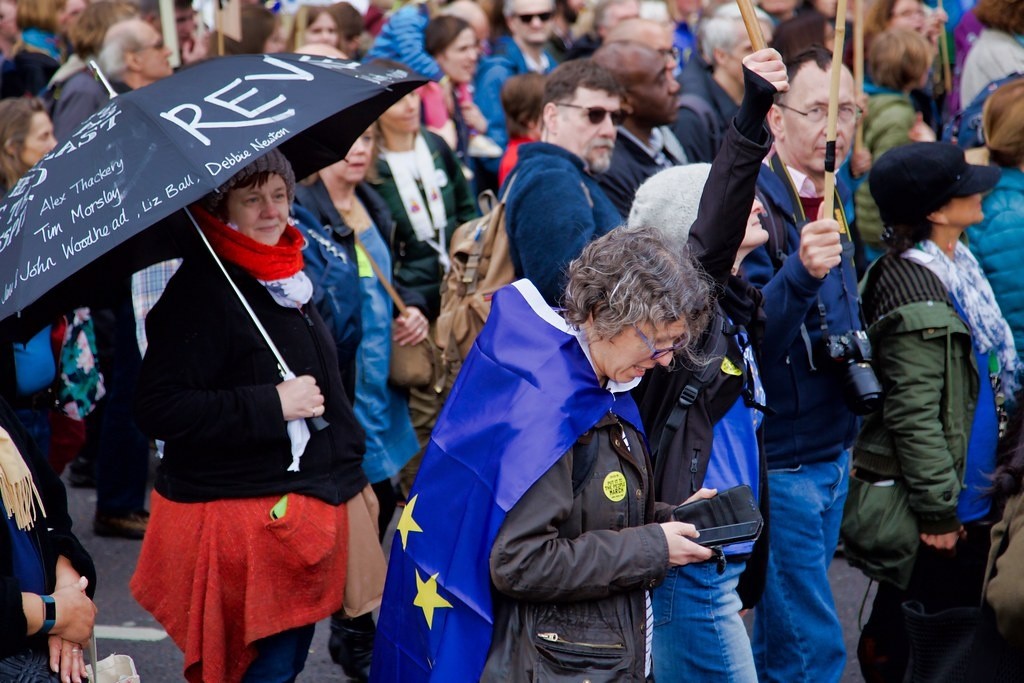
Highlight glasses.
[627,316,688,360]
[513,10,558,24]
[658,45,684,66]
[554,101,628,128]
[775,99,864,131]
[126,38,168,55]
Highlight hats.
[205,147,298,215]
[868,142,1002,216]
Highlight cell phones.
[684,520,763,546]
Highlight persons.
[0,0,1024,683]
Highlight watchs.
[40,596,56,633]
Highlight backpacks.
[434,165,594,393]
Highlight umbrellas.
[0,52,435,432]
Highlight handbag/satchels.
[904,597,1013,683]
[531,592,636,683]
[79,627,141,683]
[386,317,433,387]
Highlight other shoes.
[93,503,151,537]
[329,608,378,683]
[68,461,91,487]
[467,133,507,157]
[427,122,459,153]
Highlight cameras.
[817,329,886,416]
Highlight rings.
[71,650,79,652]
[417,328,423,334]
[313,408,315,417]
